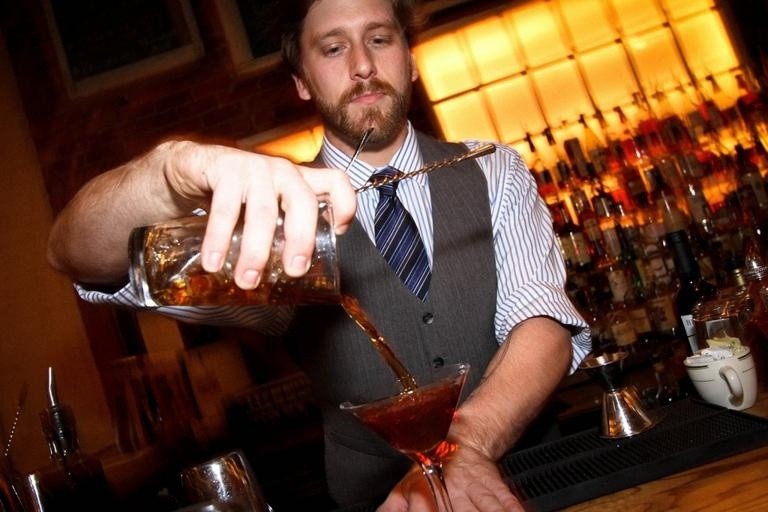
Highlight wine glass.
[339,362,471,512]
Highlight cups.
[167,448,270,511]
[683,347,758,411]
[127,193,341,309]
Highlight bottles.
[39,404,116,510]
[524,71,765,368]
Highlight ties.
[367,165,432,306]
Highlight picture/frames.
[209,0,296,80]
[40,1,207,102]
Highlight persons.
[49,0,592,512]
